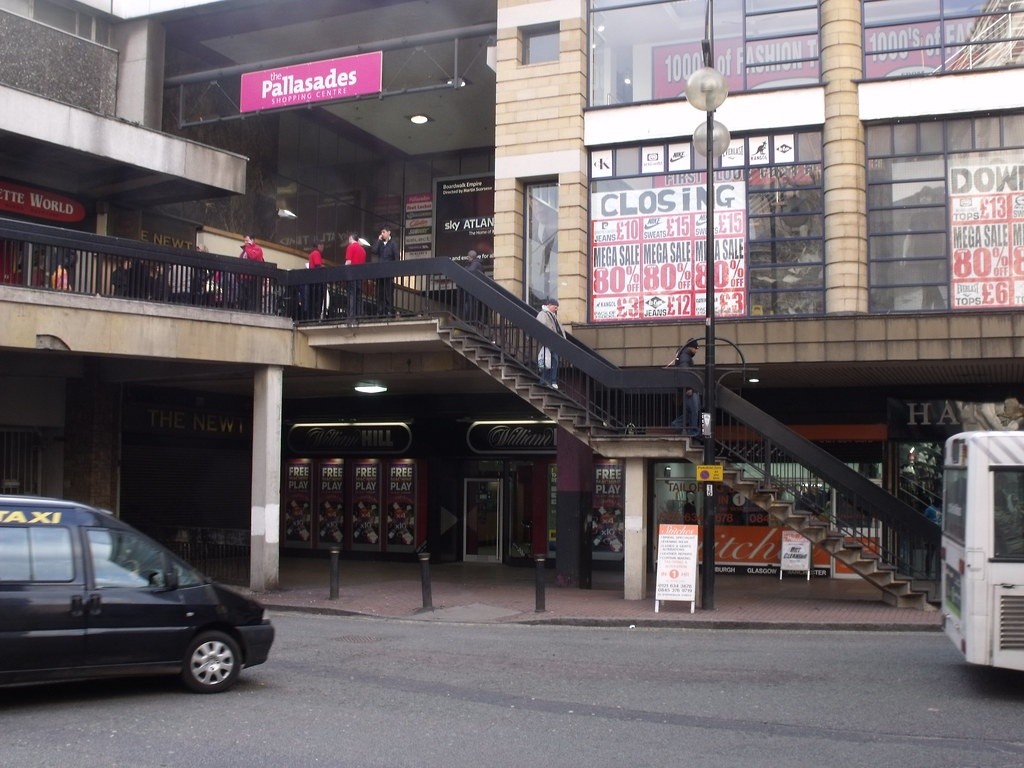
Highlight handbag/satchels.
[538,346,551,372]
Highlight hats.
[686,338,700,349]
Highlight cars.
[0,493,276,695]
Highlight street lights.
[684,38,731,613]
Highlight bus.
[938,428,1024,677]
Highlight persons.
[923,494,941,577]
[52,263,71,291]
[535,299,566,389]
[113,258,195,304]
[220,273,239,309]
[240,233,264,312]
[308,242,324,318]
[672,338,700,438]
[371,227,399,319]
[261,277,273,313]
[345,233,366,316]
[466,249,485,274]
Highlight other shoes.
[689,431,702,440]
[377,311,386,319]
[671,422,683,434]
[552,383,558,389]
[388,310,397,318]
